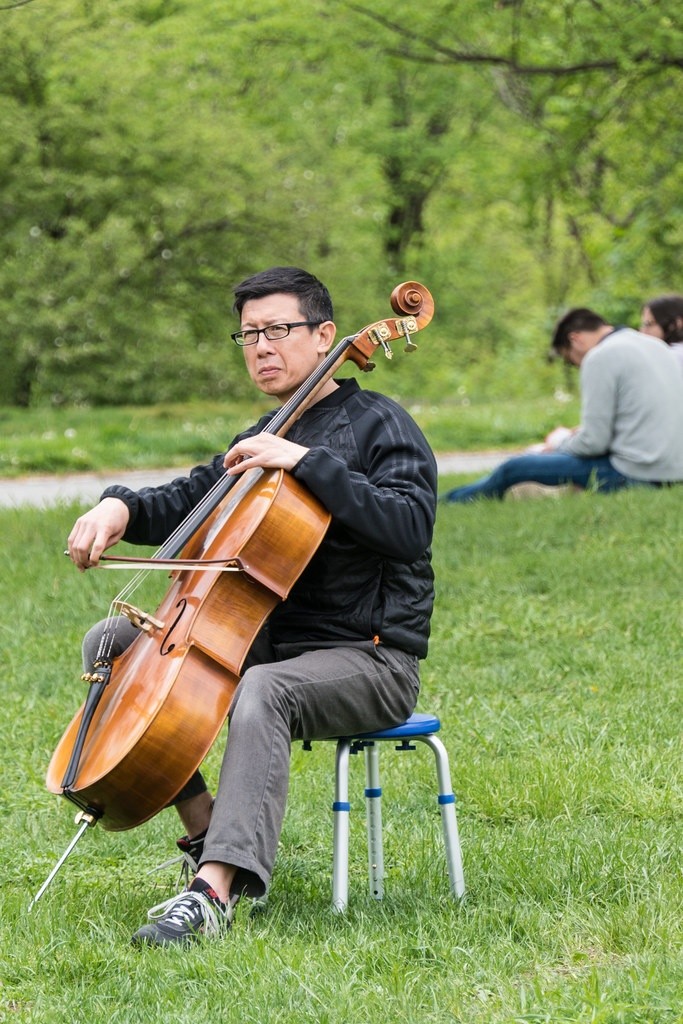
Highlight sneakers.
[130,877,239,950]
[147,797,240,909]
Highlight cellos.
[21,281,436,918]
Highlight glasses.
[564,344,574,369]
[639,322,658,329]
[230,321,321,346]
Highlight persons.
[440,296,683,504]
[68,267,438,951]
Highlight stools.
[332,712,467,914]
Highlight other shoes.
[504,482,584,503]
